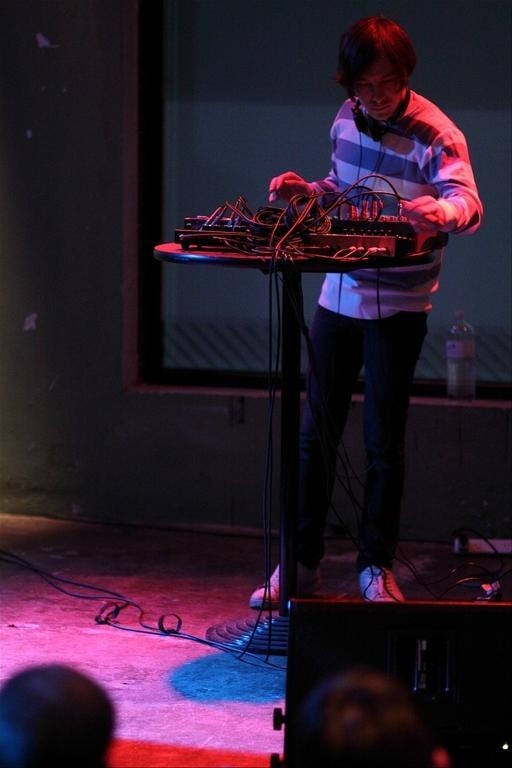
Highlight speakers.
[273,594,512,767]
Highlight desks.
[150,237,436,658]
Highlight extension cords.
[454,538,512,556]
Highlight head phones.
[352,85,411,142]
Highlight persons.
[247,17,489,612]
[302,667,431,767]
[0,664,117,768]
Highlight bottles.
[444,309,477,402]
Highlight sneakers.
[249,559,322,608]
[360,566,404,602]
